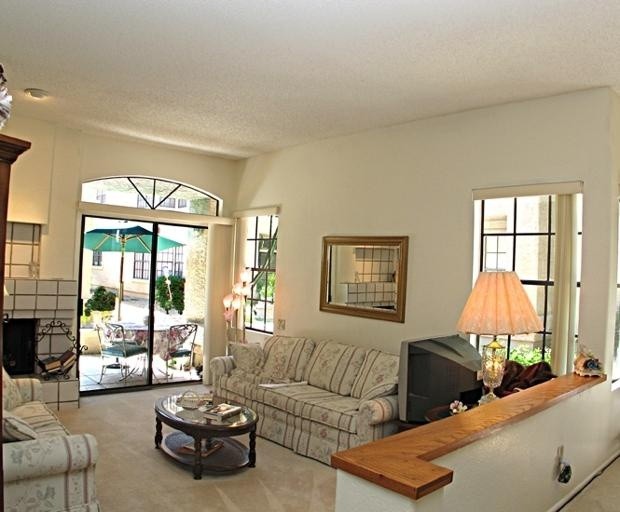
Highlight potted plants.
[88,282,116,320]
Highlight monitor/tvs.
[397,335,483,423]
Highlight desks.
[423,402,479,423]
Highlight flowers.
[450,400,467,416]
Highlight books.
[39,349,76,375]
[179,436,223,457]
[205,402,242,421]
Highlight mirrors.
[319,235,409,325]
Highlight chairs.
[95,318,197,386]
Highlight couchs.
[2,367,100,511]
[209,333,400,467]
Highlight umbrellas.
[83,225,186,320]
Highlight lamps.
[456,267,545,406]
[221,268,256,334]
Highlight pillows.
[224,337,265,373]
[301,340,361,396]
[354,374,398,411]
[347,347,402,397]
[260,332,316,383]
